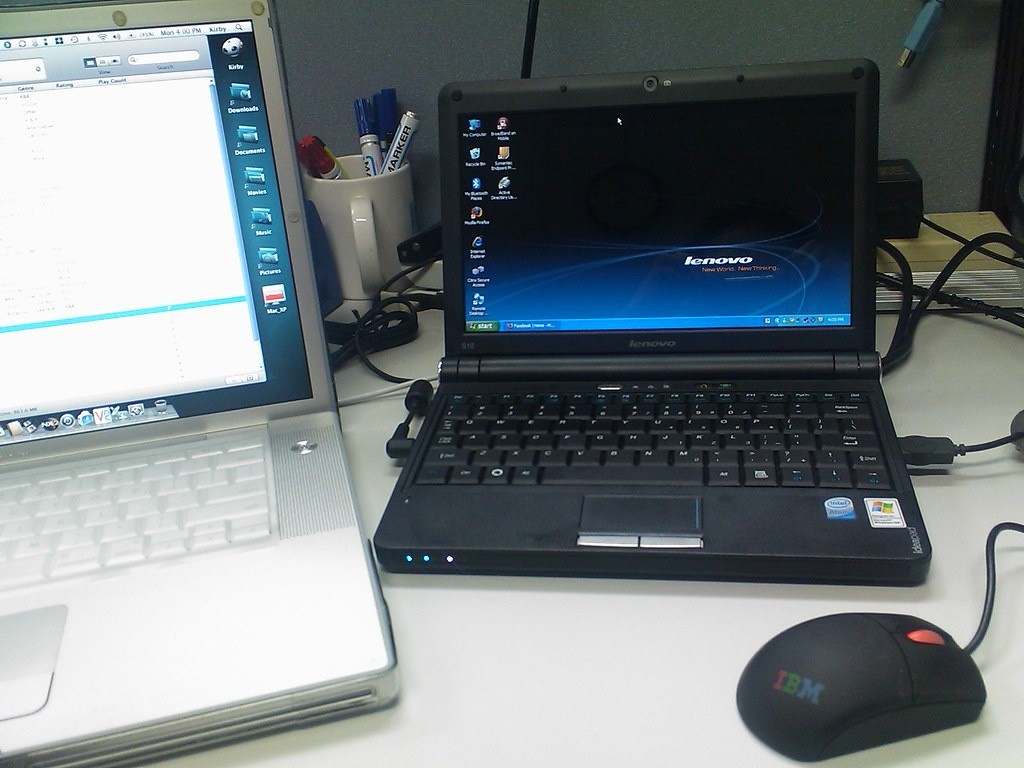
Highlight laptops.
[0,0,399,768]
[374,57,931,587]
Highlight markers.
[299,86,420,181]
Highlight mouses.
[736,612,987,763]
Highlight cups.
[302,154,417,300]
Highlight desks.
[131,260,1024,768]
